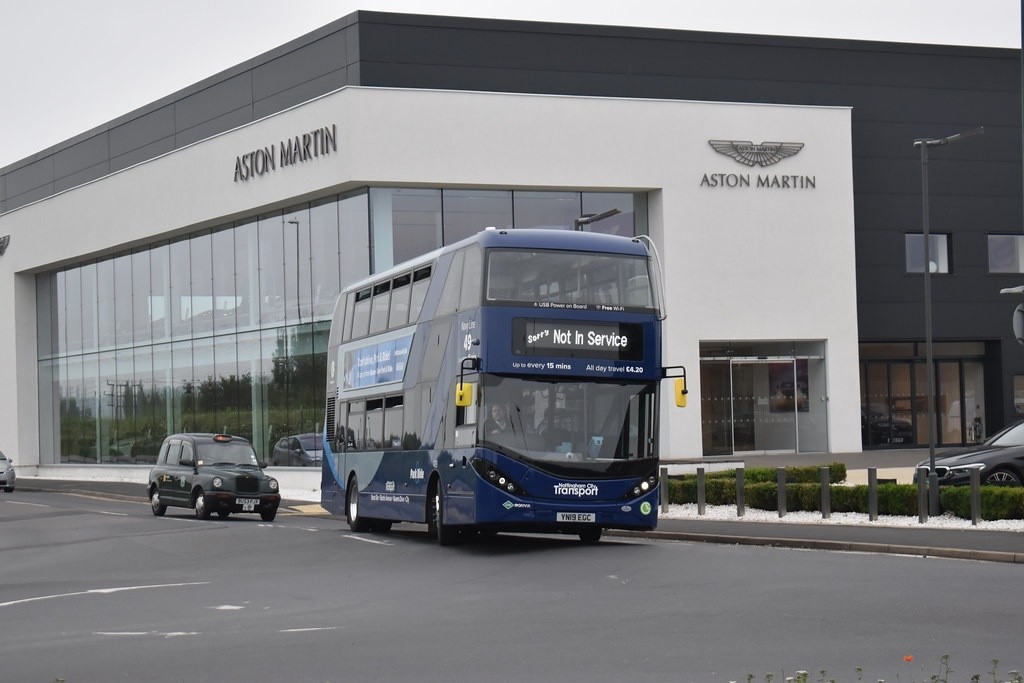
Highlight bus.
[320,229,690,548]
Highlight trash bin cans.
[877,479,896,484]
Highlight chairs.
[484,407,585,455]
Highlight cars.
[915,417,1024,487]
[0,451,17,493]
[272,433,324,467]
[147,433,281,522]
[861,405,916,447]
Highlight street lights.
[913,128,986,518]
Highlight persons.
[181,423,192,433]
[484,405,513,433]
[241,424,248,433]
[269,422,293,433]
